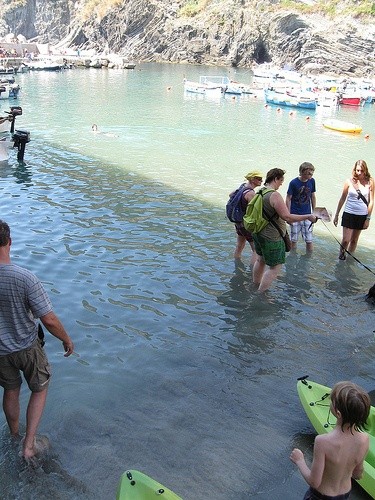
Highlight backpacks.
[243,187,276,235]
[226,183,255,223]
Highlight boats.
[0,48,135,100]
[116,469,184,500]
[323,118,363,135]
[297,374,374,500]
[177,55,375,110]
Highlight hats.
[245,171,265,178]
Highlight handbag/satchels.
[283,230,292,252]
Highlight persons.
[250,168,318,292]
[289,380,370,500]
[333,159,374,260]
[285,162,316,254]
[90,124,99,134]
[0,220,75,463]
[231,172,264,264]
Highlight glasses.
[249,176,262,182]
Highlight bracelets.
[366,216,371,220]
